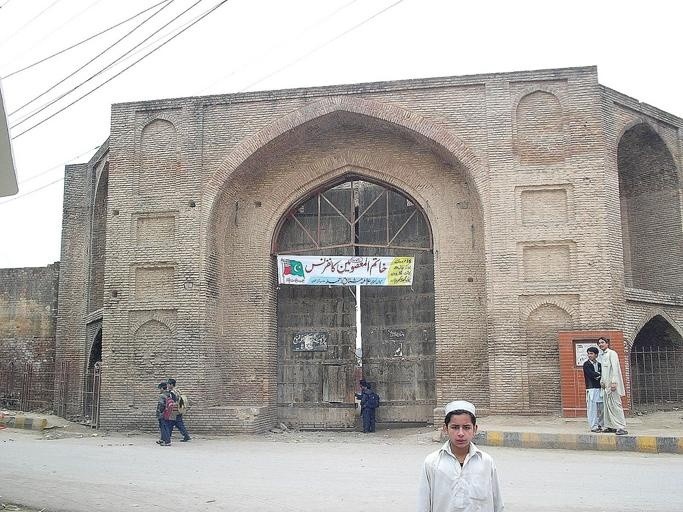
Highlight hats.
[445,400,476,416]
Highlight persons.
[168,378,192,442]
[415,400,506,512]
[355,380,376,432]
[583,347,604,432]
[155,383,177,446]
[597,337,629,434]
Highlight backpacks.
[176,391,188,414]
[366,393,379,408]
[160,395,178,421]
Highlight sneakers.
[156,439,170,446]
[180,437,190,442]
[592,425,627,434]
